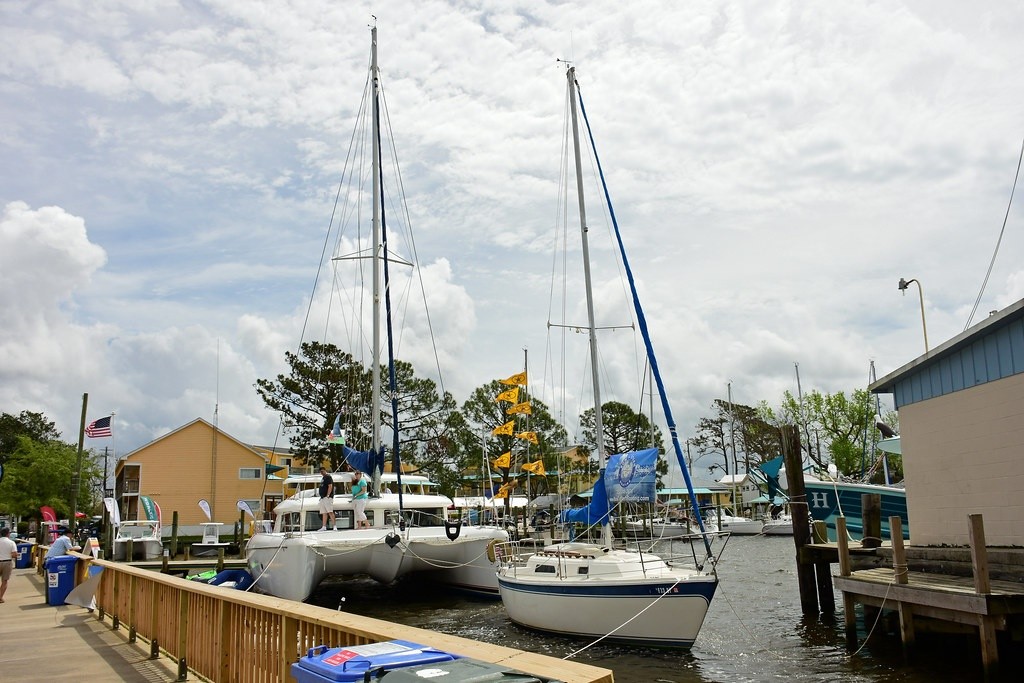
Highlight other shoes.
[0,599,4,603]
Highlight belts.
[0,560,12,562]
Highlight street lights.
[897,277,929,353]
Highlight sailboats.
[488,348,571,544]
[494,50,722,651]
[617,350,704,539]
[706,382,763,534]
[241,15,508,610]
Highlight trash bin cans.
[290,640,455,683]
[357,658,544,683]
[44,555,78,606]
[15,543,34,568]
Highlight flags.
[604,447,657,505]
[140,496,158,529]
[103,498,120,527]
[85,416,113,439]
[485,483,499,500]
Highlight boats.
[763,518,815,537]
[798,475,909,543]
[113,518,163,562]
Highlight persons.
[349,470,371,530]
[317,467,338,531]
[43,530,82,561]
[381,483,392,494]
[0,526,18,604]
